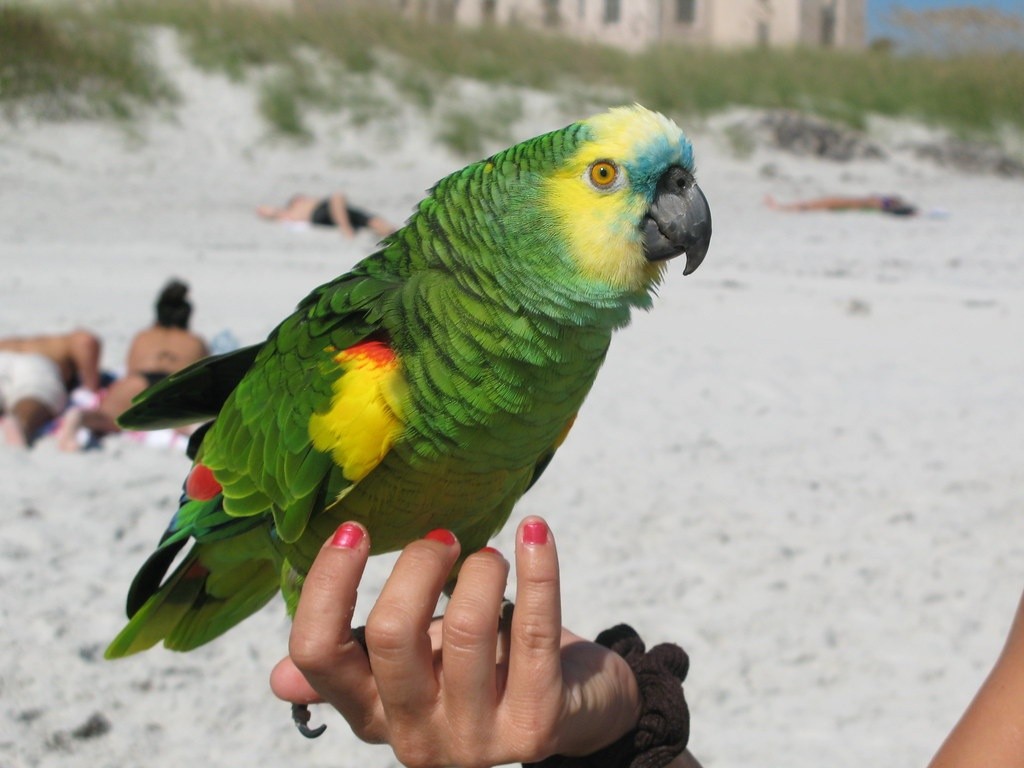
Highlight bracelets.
[521,623,691,767]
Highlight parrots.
[99,100,713,737]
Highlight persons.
[764,187,921,218]
[268,523,1023,768]
[51,275,209,454]
[255,191,402,242]
[0,326,104,448]
